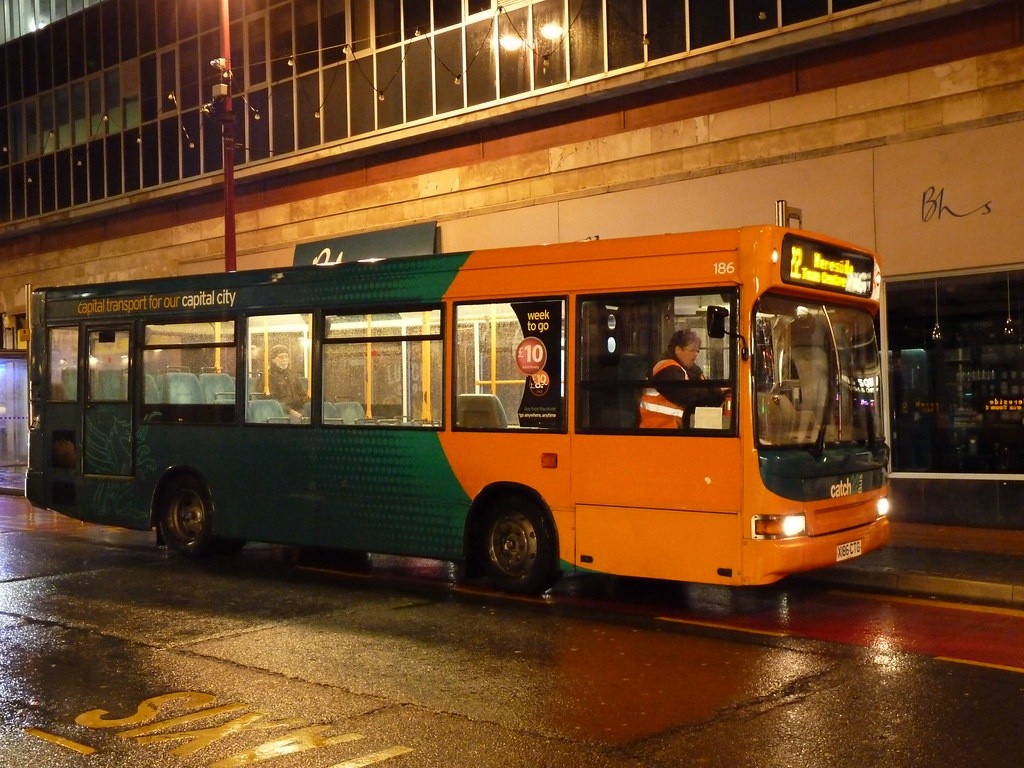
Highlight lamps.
[1004,271,1017,336]
[932,278,943,340]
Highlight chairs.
[598,351,654,429]
[457,394,507,429]
[63,367,440,428]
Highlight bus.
[26,225,895,596]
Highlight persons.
[257,344,308,423]
[638,329,731,428]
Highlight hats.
[270,345,289,360]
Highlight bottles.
[957,369,995,380]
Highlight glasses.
[683,347,700,354]
